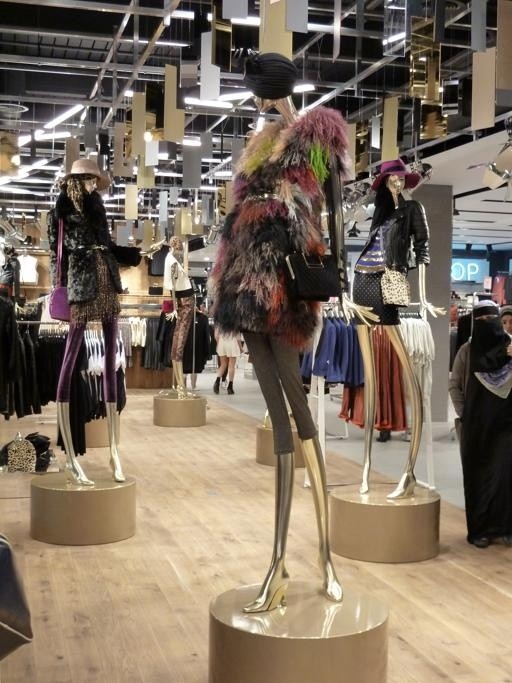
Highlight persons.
[208,50,381,616]
[161,234,198,397]
[45,157,167,486]
[498,305,512,333]
[211,323,245,395]
[447,299,512,549]
[0,245,27,308]
[350,157,450,499]
[218,332,247,390]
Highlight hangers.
[320,302,355,318]
[83,324,126,347]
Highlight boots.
[213,376,236,395]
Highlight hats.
[62,157,112,193]
[243,50,301,101]
[369,157,421,194]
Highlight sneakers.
[473,537,511,548]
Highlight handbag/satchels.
[149,284,175,315]
[48,285,73,323]
[284,250,343,304]
[380,269,413,308]
